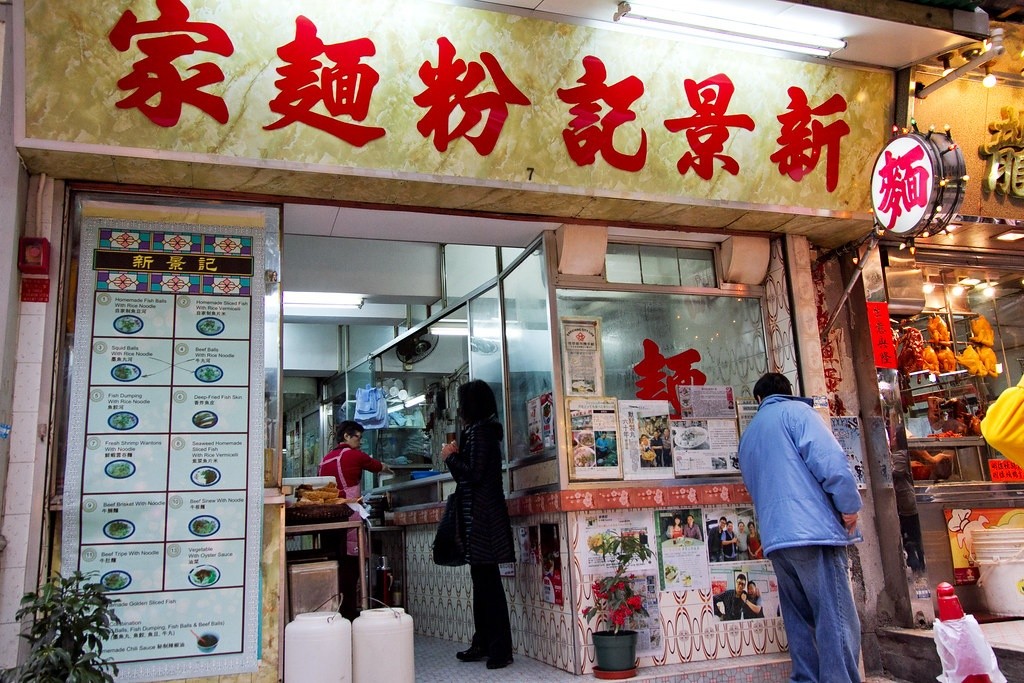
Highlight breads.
[294,482,347,505]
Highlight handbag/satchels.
[433,489,470,567]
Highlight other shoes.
[908,572,922,583]
[487,657,514,669]
[456,643,489,661]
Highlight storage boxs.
[288,559,341,619]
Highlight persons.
[639,428,672,467]
[738,372,864,683]
[665,515,684,538]
[713,573,765,621]
[432,380,518,670]
[980,373,1024,469]
[596,431,610,463]
[708,516,765,562]
[317,420,393,624]
[890,425,924,578]
[683,515,702,540]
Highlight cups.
[376,379,407,400]
[440,440,459,457]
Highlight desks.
[284,519,368,611]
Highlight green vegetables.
[116,416,130,426]
[204,320,215,331]
[193,520,213,534]
[105,576,126,587]
[121,319,134,332]
[115,368,132,379]
[109,521,129,536]
[208,569,216,583]
[201,470,208,476]
[200,367,214,379]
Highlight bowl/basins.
[571,431,596,467]
[195,631,220,653]
[911,464,952,480]
[674,426,707,449]
[411,471,441,479]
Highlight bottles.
[392,577,403,606]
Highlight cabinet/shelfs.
[376,422,439,499]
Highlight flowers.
[581,525,652,635]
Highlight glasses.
[353,434,362,440]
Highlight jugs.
[377,555,393,603]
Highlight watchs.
[744,599,748,603]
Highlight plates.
[99,313,226,591]
[542,403,553,425]
[684,540,693,545]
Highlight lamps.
[282,297,365,309]
[923,267,995,298]
[613,1,849,61]
[935,52,955,77]
[979,60,1001,90]
[426,322,524,336]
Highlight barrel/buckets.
[284,593,352,683]
[969,529,1024,617]
[352,596,415,683]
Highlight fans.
[472,334,499,353]
[395,327,440,371]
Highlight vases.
[591,629,639,671]
